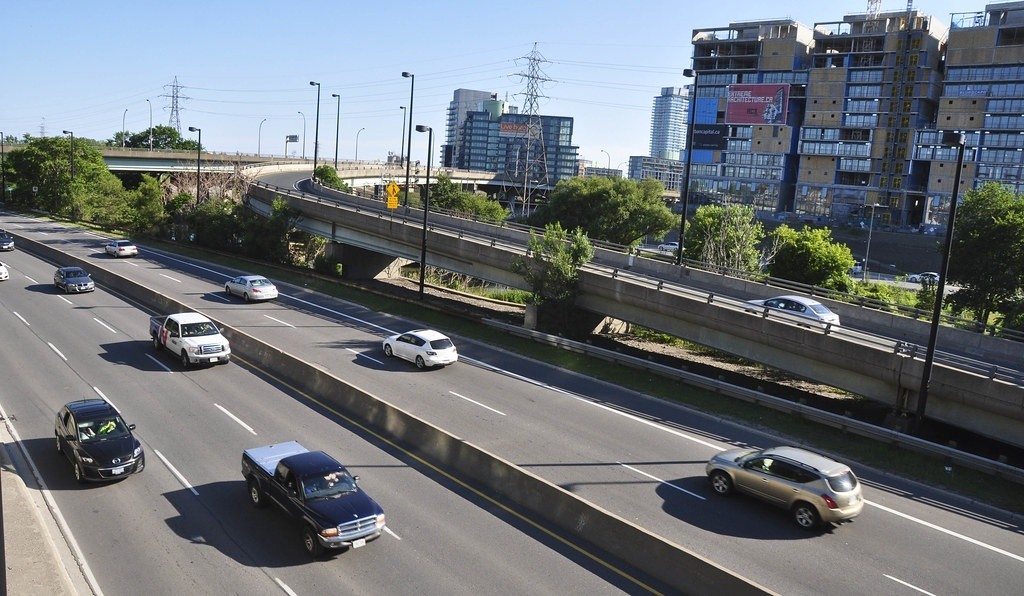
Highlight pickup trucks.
[241,440,386,557]
[149,312,231,370]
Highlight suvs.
[706,446,864,532]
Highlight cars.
[658,242,679,251]
[55,399,145,483]
[225,275,278,303]
[907,272,948,286]
[848,262,869,275]
[745,296,841,334]
[382,329,459,370]
[676,69,698,266]
[0,262,9,281]
[0,232,15,251]
[105,239,139,258]
[53,267,95,294]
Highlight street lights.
[356,127,365,160]
[122,109,128,151]
[601,149,610,176]
[298,111,305,160]
[189,127,201,204]
[147,100,152,152]
[913,133,965,437]
[332,93,341,170]
[310,81,320,178]
[416,125,432,301]
[63,130,74,222]
[259,118,266,158]
[399,71,415,206]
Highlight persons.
[79,427,92,440]
[97,420,117,436]
[324,473,339,489]
[198,323,213,332]
[304,477,321,493]
